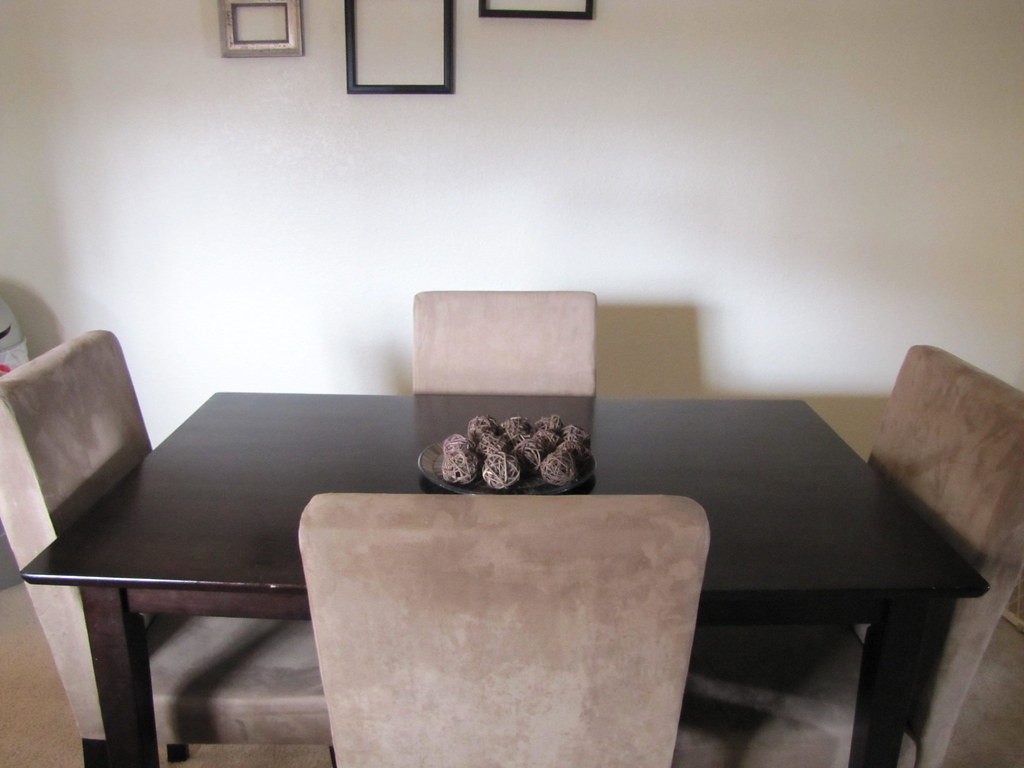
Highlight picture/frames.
[478,0,593,20]
[217,0,303,57]
[344,0,455,94]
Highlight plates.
[418,441,598,495]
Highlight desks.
[17,391,991,768]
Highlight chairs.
[410,289,598,396]
[0,329,336,768]
[298,493,709,768]
[672,346,1024,768]
